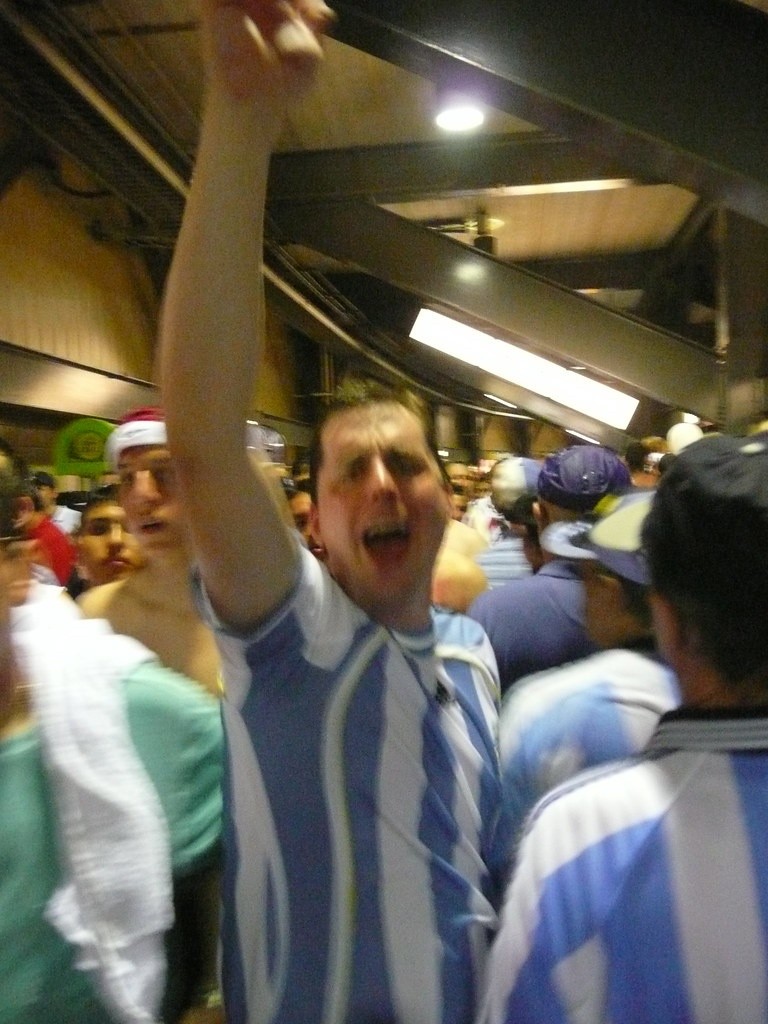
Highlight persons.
[0,408,768,1024]
[160,0,501,1024]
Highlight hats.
[488,454,544,515]
[641,432,768,605]
[539,491,663,584]
[539,445,633,508]
[102,403,169,470]
[29,471,56,488]
